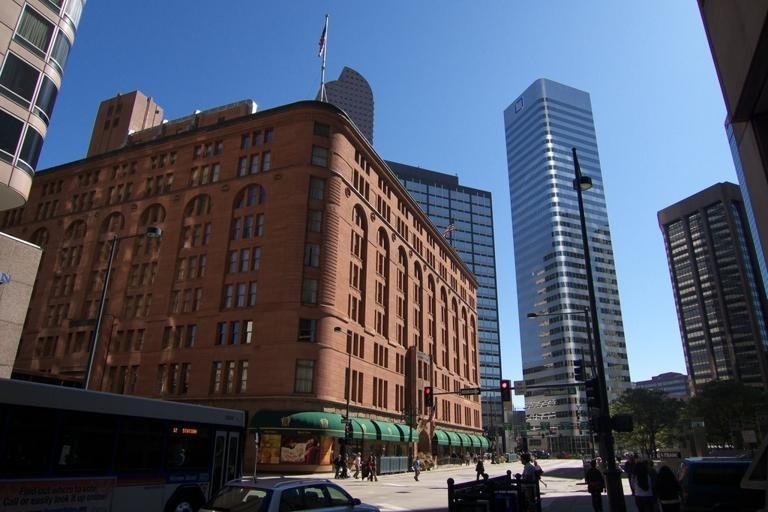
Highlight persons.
[333,452,377,481]
[472,451,478,464]
[465,451,470,466]
[624,453,684,512]
[533,461,547,489]
[491,452,495,464]
[521,454,535,479]
[451,450,457,465]
[586,460,605,512]
[414,456,420,481]
[475,455,485,480]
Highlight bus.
[0,378,248,512]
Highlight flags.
[318,23,327,57]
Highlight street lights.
[527,147,628,511]
[82,226,162,390]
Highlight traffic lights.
[424,387,433,407]
[500,379,512,402]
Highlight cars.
[198,477,380,512]
